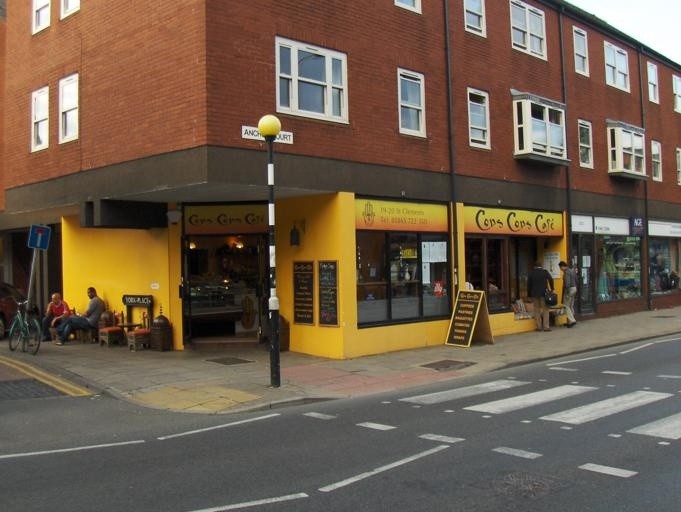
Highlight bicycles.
[8,294,41,356]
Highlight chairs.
[98,309,150,352]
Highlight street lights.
[257,113,282,389]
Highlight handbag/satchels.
[554,314,568,326]
[545,294,557,305]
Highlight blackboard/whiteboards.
[293,260,339,326]
[445,290,483,348]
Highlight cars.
[0,281,40,341]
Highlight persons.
[41,293,70,342]
[55,287,105,345]
[526,261,554,331]
[488,281,498,291]
[559,261,578,328]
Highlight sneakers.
[40,328,64,346]
[564,322,576,328]
[536,328,552,332]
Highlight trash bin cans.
[150,314,172,351]
